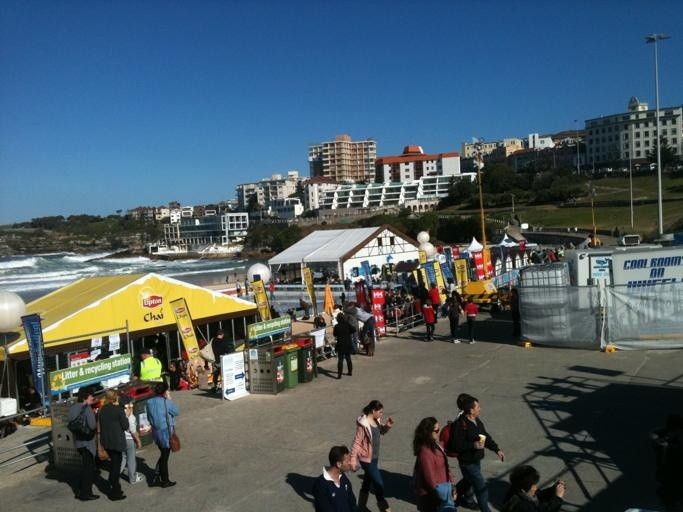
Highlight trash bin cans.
[266,338,313,394]
[118,384,155,447]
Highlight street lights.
[473,138,493,279]
[647,31,671,236]
[574,119,580,176]
[590,188,597,248]
[548,148,559,171]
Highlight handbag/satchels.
[97,445,111,462]
[168,429,181,453]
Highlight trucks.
[615,233,641,246]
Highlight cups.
[479,435,488,447]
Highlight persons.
[532,239,576,264]
[343,301,376,357]
[333,311,356,378]
[22,386,42,416]
[384,277,480,344]
[161,361,179,390]
[211,328,233,393]
[235,277,352,320]
[313,392,567,512]
[67,382,181,500]
[510,288,521,328]
[134,347,169,382]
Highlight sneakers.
[148,481,177,488]
[78,494,101,501]
[105,483,127,502]
[130,473,144,485]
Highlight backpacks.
[67,403,96,442]
[440,417,467,458]
